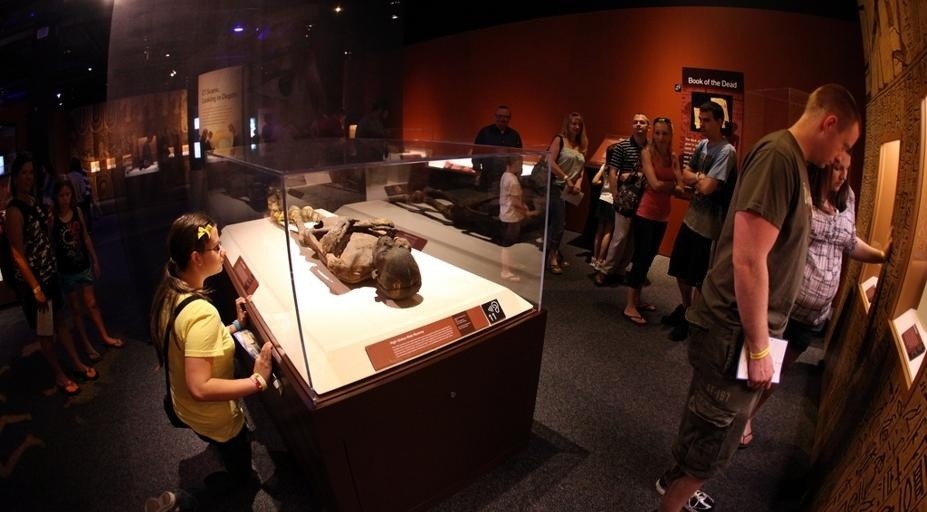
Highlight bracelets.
[248,374,267,393]
[695,172,701,180]
[750,346,770,359]
[233,319,242,332]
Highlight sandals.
[550,262,562,274]
[561,259,570,271]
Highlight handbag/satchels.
[528,161,551,187]
[164,393,190,429]
[613,174,646,217]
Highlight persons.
[473,101,737,340]
[145,213,274,512]
[201,123,238,149]
[654,83,861,512]
[738,153,894,450]
[267,195,422,300]
[0,155,123,394]
[141,136,154,164]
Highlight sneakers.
[590,257,597,266]
[668,325,688,340]
[662,304,685,324]
[144,491,175,512]
[596,271,605,283]
[655,475,715,511]
[595,258,605,270]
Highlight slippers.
[623,313,646,325]
[739,431,753,447]
[637,302,655,310]
[90,351,102,361]
[502,271,520,282]
[59,377,80,394]
[82,365,99,380]
[110,338,123,346]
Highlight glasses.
[655,118,671,123]
[206,241,222,251]
[493,114,510,119]
